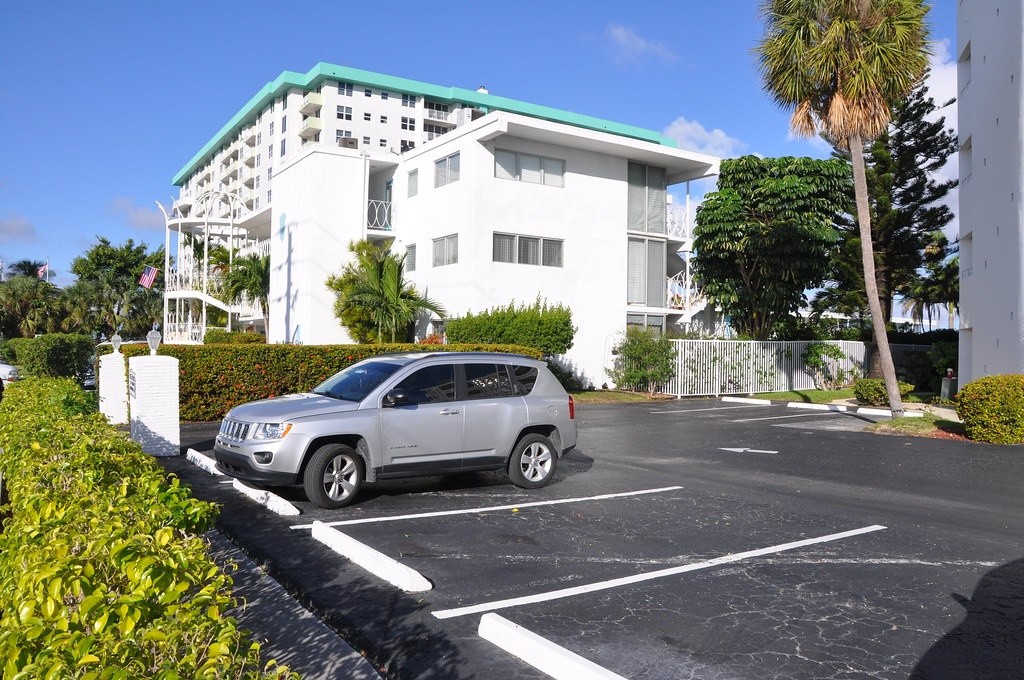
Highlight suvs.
[213,352,579,510]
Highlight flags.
[38,263,48,279]
[139,266,157,290]
